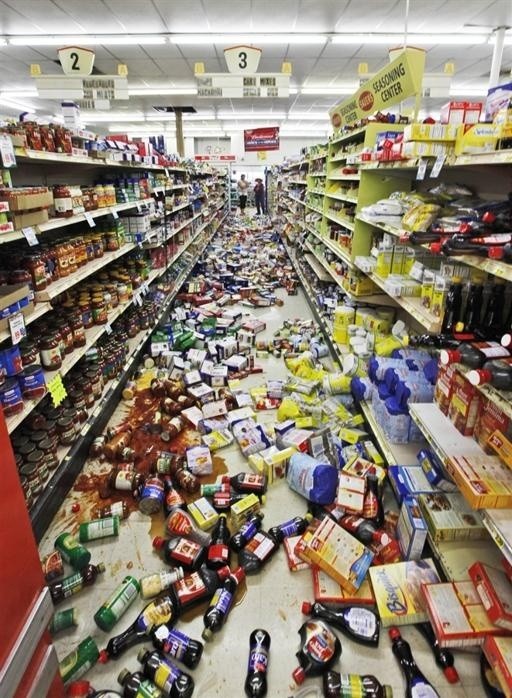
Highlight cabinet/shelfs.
[1,138,231,554]
[284,118,511,698]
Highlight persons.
[236,173,250,216]
[253,178,265,215]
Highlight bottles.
[91,377,193,469]
[38,234,78,285]
[400,197,511,266]
[49,471,460,698]
[411,275,511,391]
[52,179,116,219]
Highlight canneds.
[0,121,335,582]
